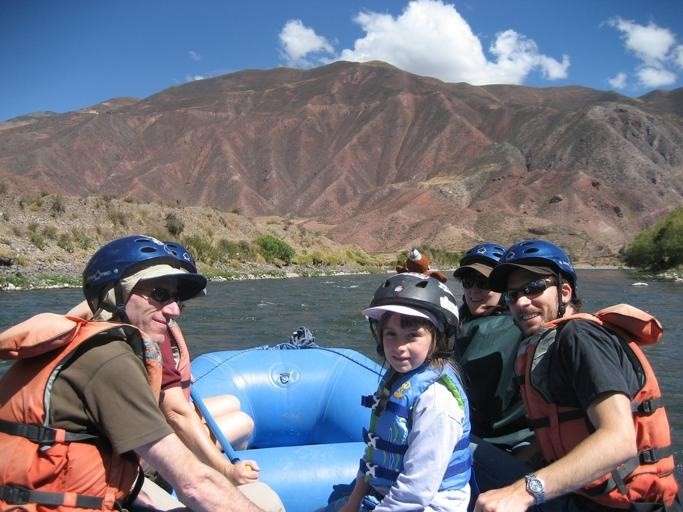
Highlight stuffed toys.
[394,249,448,284]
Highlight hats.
[488,261,565,292]
[91,261,207,322]
[360,303,448,335]
[454,256,497,279]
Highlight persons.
[452,243,543,446]
[0,237,284,512]
[313,271,470,512]
[65,243,260,487]
[474,239,678,512]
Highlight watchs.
[522,471,545,505]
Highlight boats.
[171,346,402,511]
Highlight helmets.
[497,240,577,282]
[83,235,180,314]
[370,272,461,338]
[162,241,197,271]
[461,244,508,262]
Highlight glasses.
[502,278,563,306]
[459,274,491,290]
[132,287,180,304]
